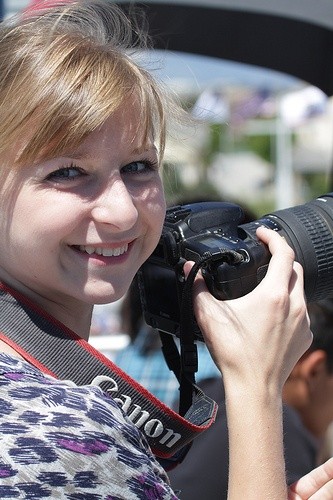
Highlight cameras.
[137,193,333,342]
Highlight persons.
[167,336,333,500]
[0,0,333,500]
